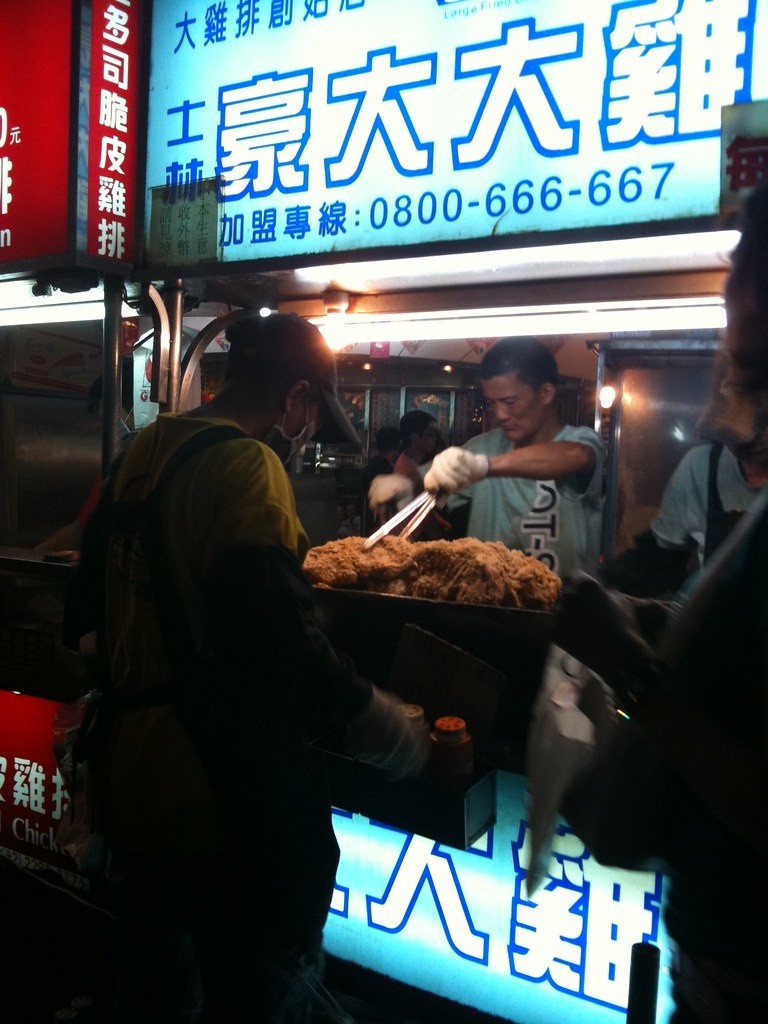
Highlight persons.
[63,316,439,1024]
[32,471,101,550]
[355,337,615,599]
[526,173,768,1024]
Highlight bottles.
[387,704,433,764]
[427,717,474,819]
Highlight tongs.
[360,487,439,551]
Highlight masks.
[120,405,133,438]
[263,393,313,465]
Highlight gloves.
[424,445,488,509]
[369,473,413,511]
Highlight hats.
[695,351,768,442]
[226,311,364,448]
